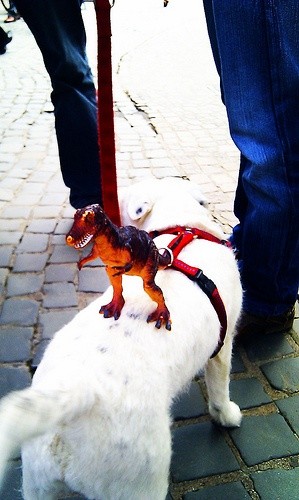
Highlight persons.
[203,0,299,340]
[11,0,103,207]
[5,0,21,23]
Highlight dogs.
[0,176,244,500]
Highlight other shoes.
[234,310,295,338]
